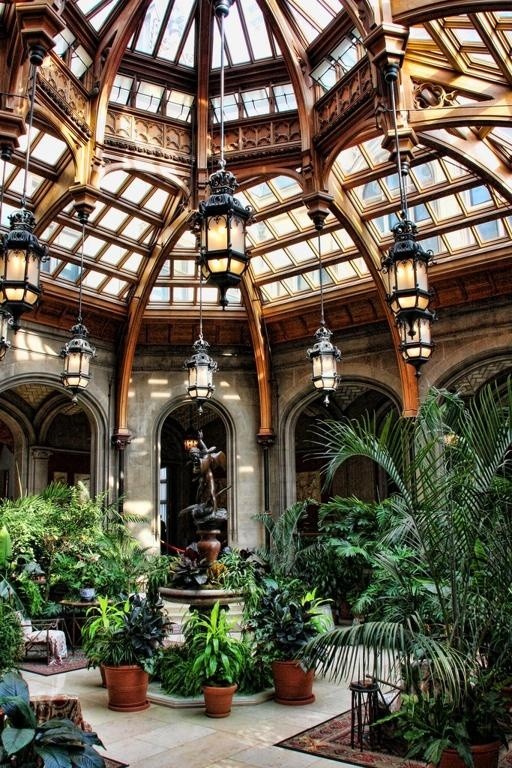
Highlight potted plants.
[76,555,103,600]
[250,580,318,707]
[323,380,509,766]
[91,592,166,714]
[185,611,242,720]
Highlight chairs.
[18,618,64,666]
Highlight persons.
[179,429,232,531]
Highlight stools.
[347,675,379,755]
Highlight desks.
[60,596,113,656]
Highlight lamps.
[306,219,343,408]
[0,44,47,335]
[60,211,98,407]
[182,237,220,417]
[190,0,257,311]
[375,63,438,341]
[396,161,438,382]
[0,145,13,362]
